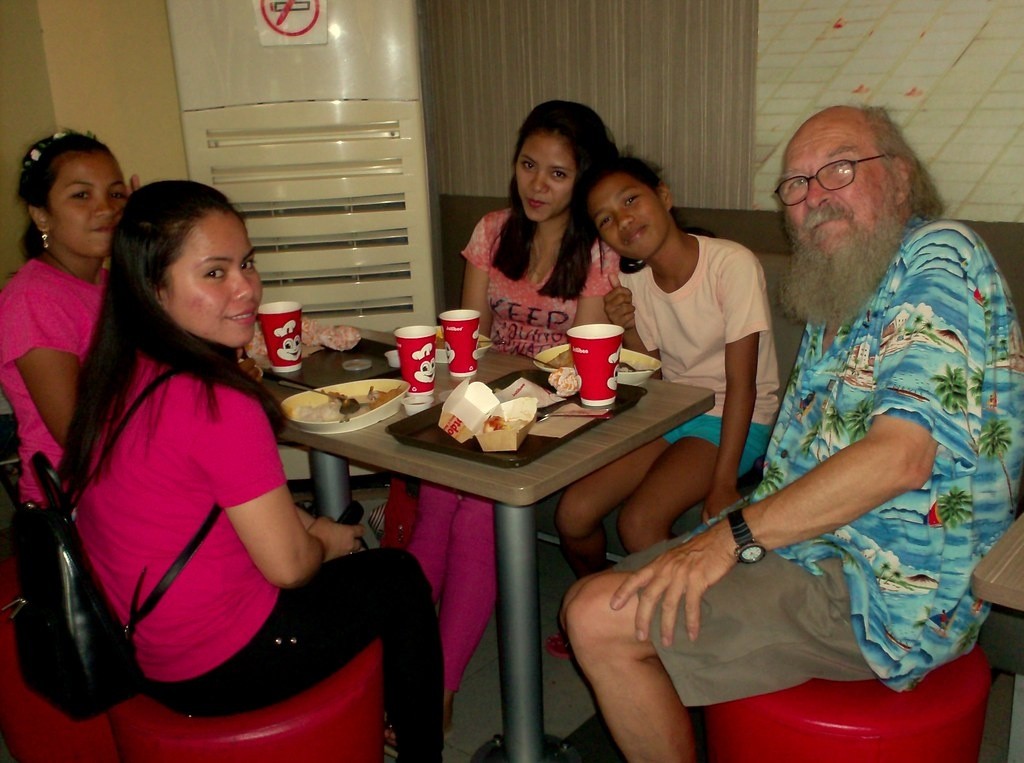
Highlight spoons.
[533,408,612,423]
[278,380,360,415]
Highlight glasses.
[771,151,887,209]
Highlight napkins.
[528,402,607,440]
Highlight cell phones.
[335,500,365,526]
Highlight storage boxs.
[437,377,538,452]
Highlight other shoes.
[545,628,576,660]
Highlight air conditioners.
[166,1,442,333]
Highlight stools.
[706,644,991,763]
[0,555,383,763]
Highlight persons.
[385,98,778,763]
[0,127,442,763]
[558,105,1024,763]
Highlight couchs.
[442,195,1024,673]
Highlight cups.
[567,324,624,406]
[438,309,481,378]
[394,324,436,397]
[257,301,303,373]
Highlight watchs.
[727,507,768,567]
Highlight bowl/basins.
[533,341,661,386]
[401,395,433,416]
[384,350,401,367]
[281,379,411,434]
[432,325,494,364]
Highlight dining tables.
[228,324,714,763]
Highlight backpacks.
[0,362,280,725]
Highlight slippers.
[382,719,457,759]
